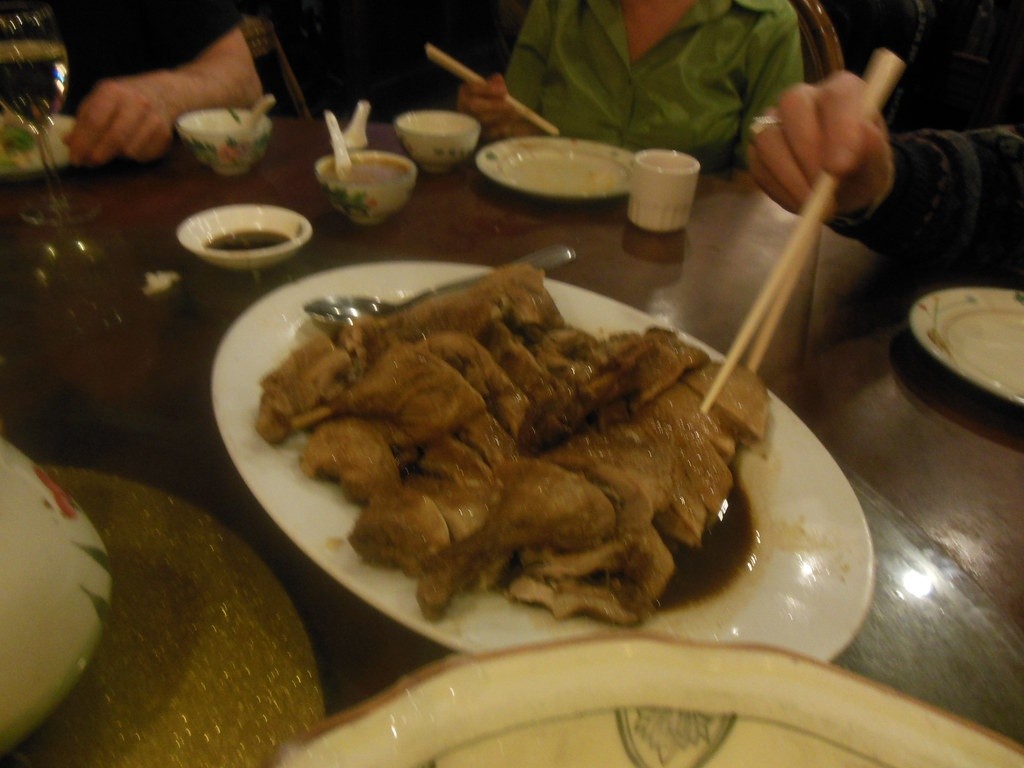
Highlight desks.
[0,113,1024,766]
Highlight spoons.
[342,101,371,147]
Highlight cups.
[627,148,700,231]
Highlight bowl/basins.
[395,110,480,173]
[313,151,417,225]
[175,106,271,170]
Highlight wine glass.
[0,5,100,222]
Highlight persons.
[455,2,805,175]
[746,71,1024,287]
[1,1,262,167]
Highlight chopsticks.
[426,43,559,135]
[700,47,903,411]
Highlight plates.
[0,108,76,179]
[909,287,1024,406]
[0,465,112,757]
[212,261,874,666]
[176,202,312,269]
[252,633,1024,768]
[474,138,636,198]
